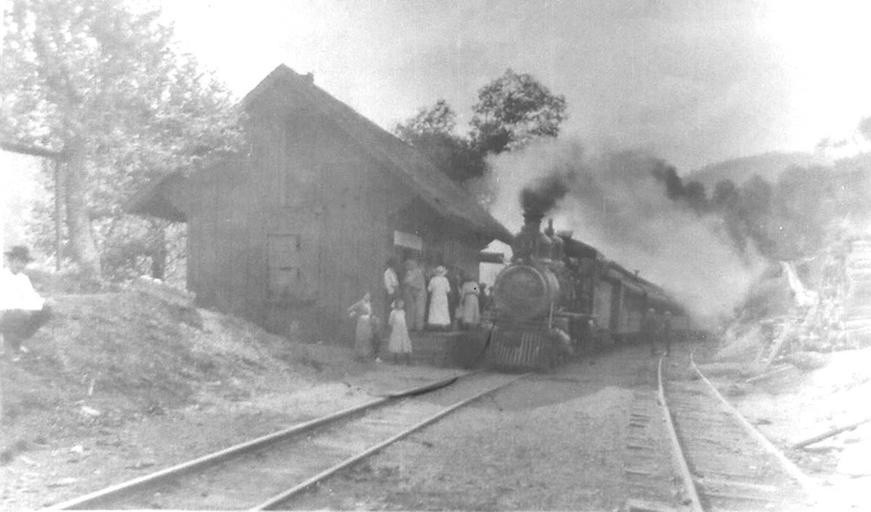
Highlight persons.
[643,309,657,356]
[662,311,671,356]
[347,254,487,365]
[0,247,47,362]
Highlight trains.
[453,208,709,373]
